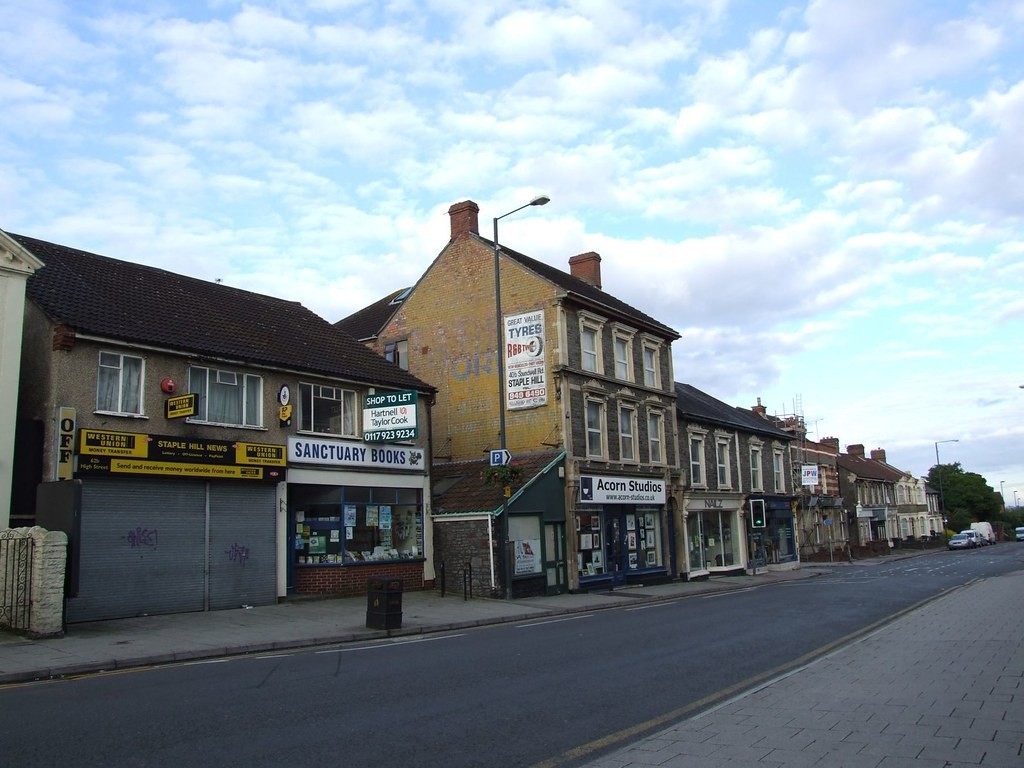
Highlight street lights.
[491,196,552,599]
[934,439,959,540]
[1000,481,1005,510]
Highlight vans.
[1015,527,1024,541]
[970,522,996,545]
[960,529,982,548]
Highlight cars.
[948,533,973,550]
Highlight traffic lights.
[750,499,766,529]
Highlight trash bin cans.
[365,575,403,630]
[891,537,903,550]
